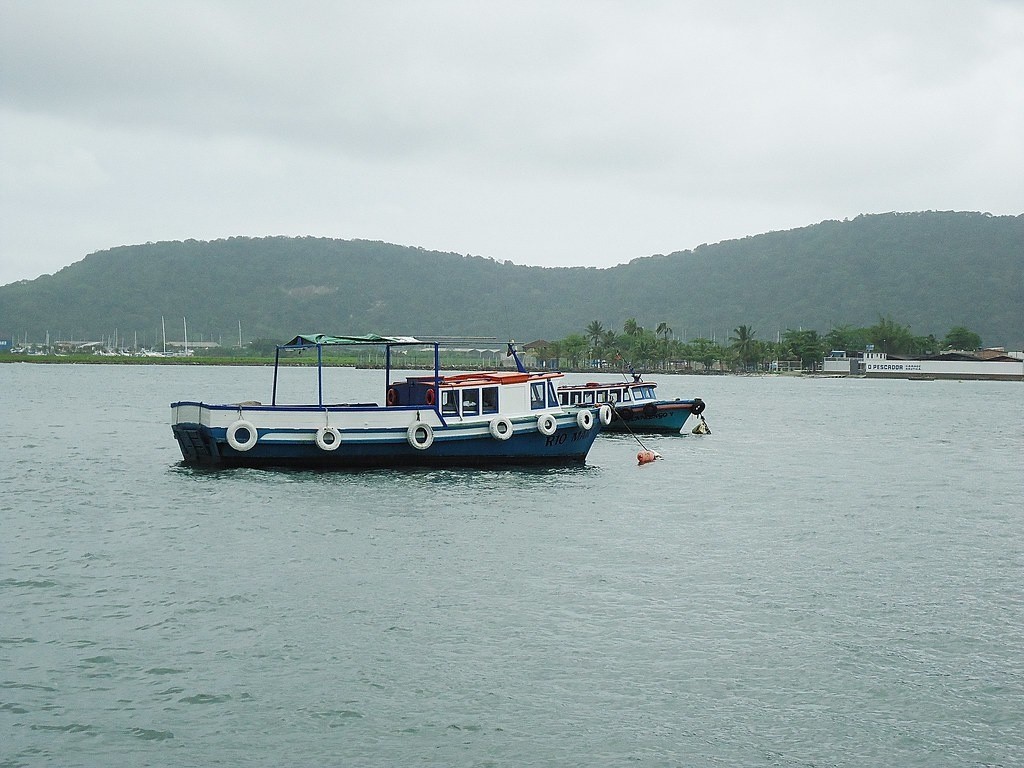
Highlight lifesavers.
[425,389,435,405]
[692,402,705,415]
[387,389,399,406]
[577,410,593,430]
[225,419,258,451]
[599,405,611,425]
[643,403,658,417]
[407,421,434,450]
[489,415,514,441]
[315,426,342,451]
[620,407,632,420]
[537,414,557,436]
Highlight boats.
[909,375,936,382]
[169,333,617,472]
[554,378,710,438]
[865,358,1024,382]
